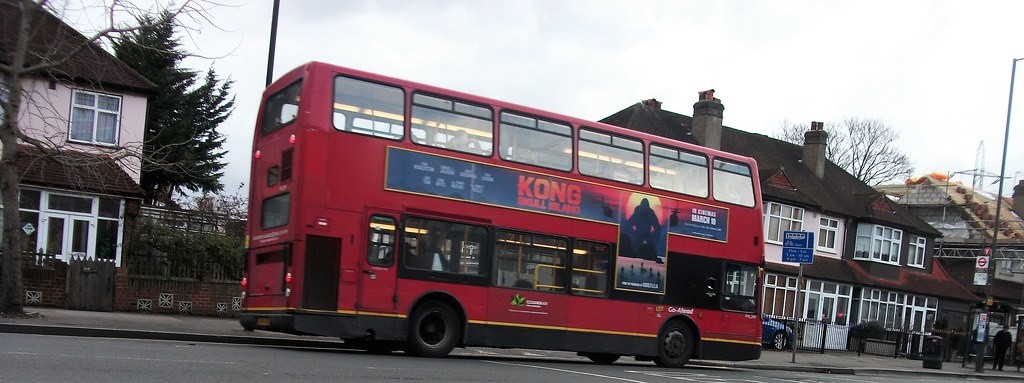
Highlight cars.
[762,316,793,351]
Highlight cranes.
[953,141,1013,193]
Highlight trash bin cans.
[923,335,944,369]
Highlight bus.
[234,62,766,369]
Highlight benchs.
[962,339,996,369]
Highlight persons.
[992,324,1012,371]
[1015,329,1024,366]
[973,327,987,373]
